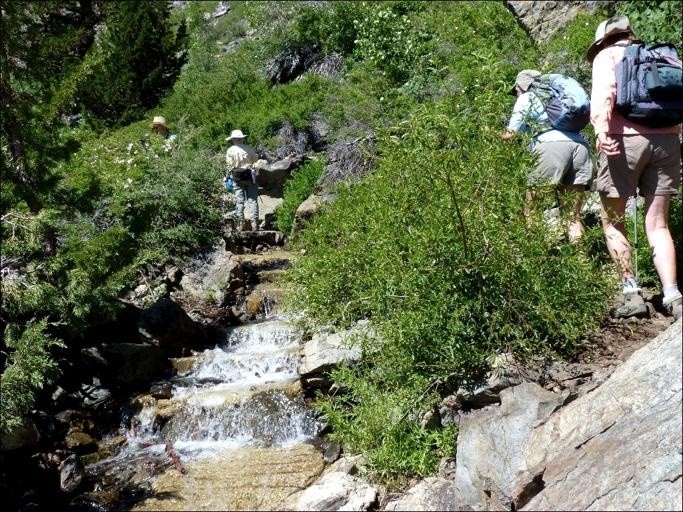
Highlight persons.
[498,68,596,259]
[225,129,260,232]
[149,116,175,140]
[585,14,682,323]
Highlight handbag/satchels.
[232,167,252,181]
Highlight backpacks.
[528,74,591,135]
[605,38,682,130]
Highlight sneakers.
[609,291,648,317]
[662,294,682,320]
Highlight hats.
[587,16,638,62]
[508,70,542,97]
[224,130,247,141]
[149,116,170,130]
